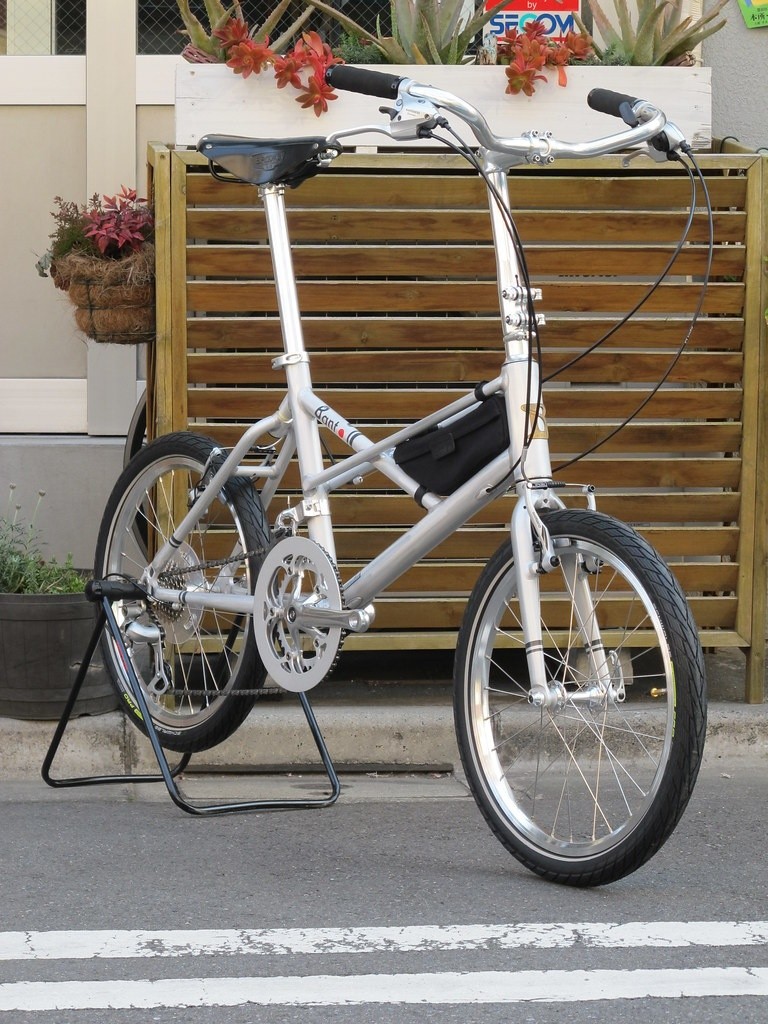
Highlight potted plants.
[2,482,127,722]
[175,0,732,153]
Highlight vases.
[54,238,153,345]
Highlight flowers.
[28,182,151,278]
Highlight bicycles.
[41,66,709,887]
